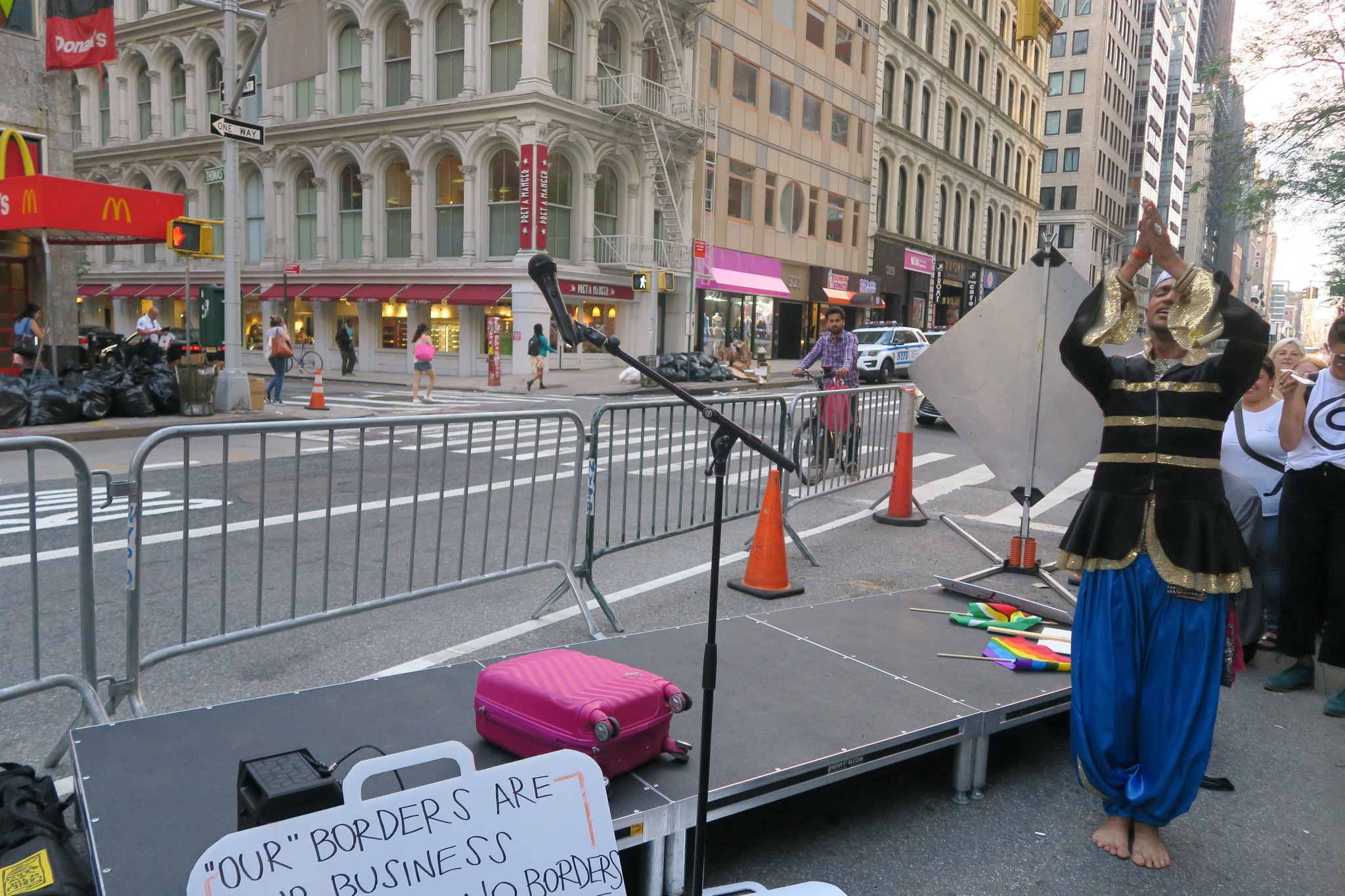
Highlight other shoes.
[1265,662,1316,694]
[809,454,824,469]
[846,464,860,479]
[424,395,436,402]
[539,384,547,390]
[412,398,422,404]
[1325,684,1345,717]
[526,381,532,391]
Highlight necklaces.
[1153,358,1182,373]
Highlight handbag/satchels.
[271,328,294,358]
[414,336,434,362]
[11,319,38,359]
[822,373,853,432]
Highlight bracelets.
[1115,248,1150,306]
[1172,262,1199,295]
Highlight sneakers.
[272,399,285,405]
[263,391,272,403]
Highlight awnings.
[822,287,886,308]
[76,283,511,305]
[706,267,790,298]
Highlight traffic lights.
[166,219,203,254]
[631,272,651,291]
[657,271,675,290]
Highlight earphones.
[843,322,844,326]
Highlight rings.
[1279,380,1284,384]
[1137,221,1143,230]
[1154,223,1162,235]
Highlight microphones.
[527,253,578,346]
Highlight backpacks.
[527,336,545,356]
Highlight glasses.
[1326,346,1345,365]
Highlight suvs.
[850,320,955,425]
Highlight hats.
[1152,267,1175,285]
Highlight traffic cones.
[725,469,806,600]
[304,371,331,411]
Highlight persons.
[411,322,436,403]
[338,317,357,376]
[1220,315,1345,720]
[704,312,767,354]
[527,323,558,392]
[135,306,169,344]
[297,327,304,335]
[263,315,291,405]
[1058,198,1270,867]
[15,303,50,368]
[792,306,861,480]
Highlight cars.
[99,326,226,365]
[77,323,125,365]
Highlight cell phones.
[1279,369,1317,386]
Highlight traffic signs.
[208,112,266,147]
[219,74,257,102]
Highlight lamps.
[575,307,616,317]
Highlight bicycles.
[790,364,861,487]
[286,339,323,374]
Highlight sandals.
[1257,634,1279,650]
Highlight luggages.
[474,648,692,790]
